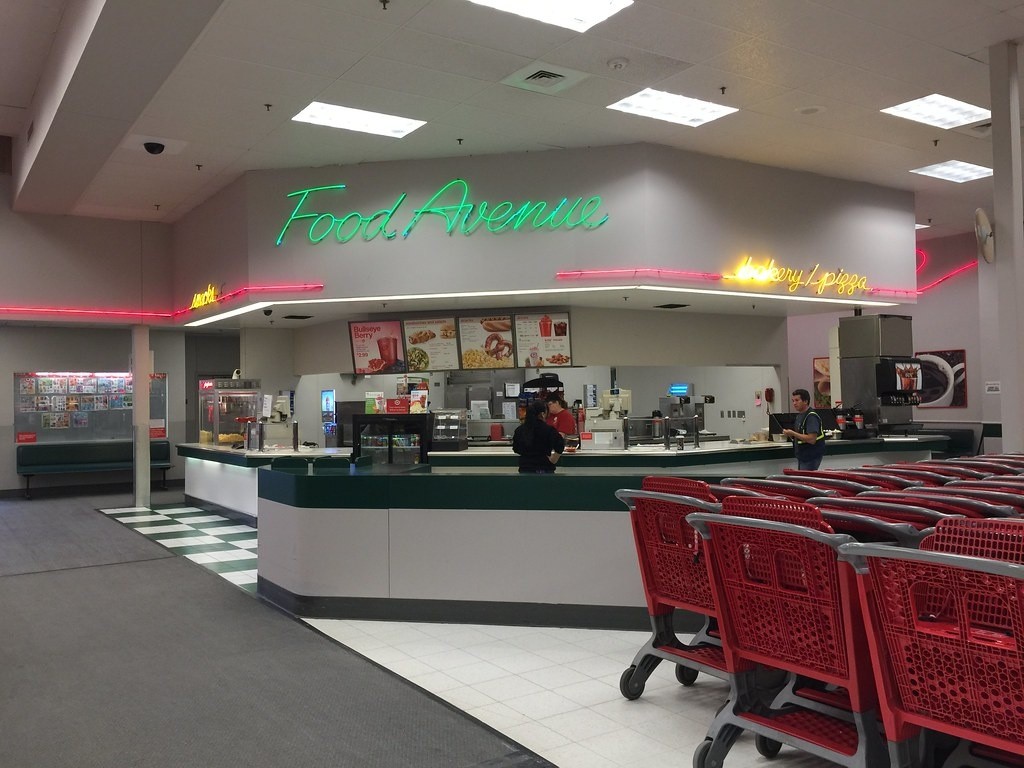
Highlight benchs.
[919,429,975,460]
[16,440,176,499]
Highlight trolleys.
[614,452,1024,768]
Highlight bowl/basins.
[771,434,788,442]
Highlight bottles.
[326,396,330,409]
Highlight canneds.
[362,434,420,447]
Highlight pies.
[408,330,436,343]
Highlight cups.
[375,398,384,410]
[377,337,398,366]
[916,355,965,407]
[539,320,552,336]
[901,378,917,390]
[676,436,684,450]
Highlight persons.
[783,389,826,470]
[512,400,565,474]
[544,393,576,434]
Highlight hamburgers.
[440,324,455,339]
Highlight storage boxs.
[772,434,788,442]
[830,432,843,440]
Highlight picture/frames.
[813,357,831,409]
[915,349,968,409]
